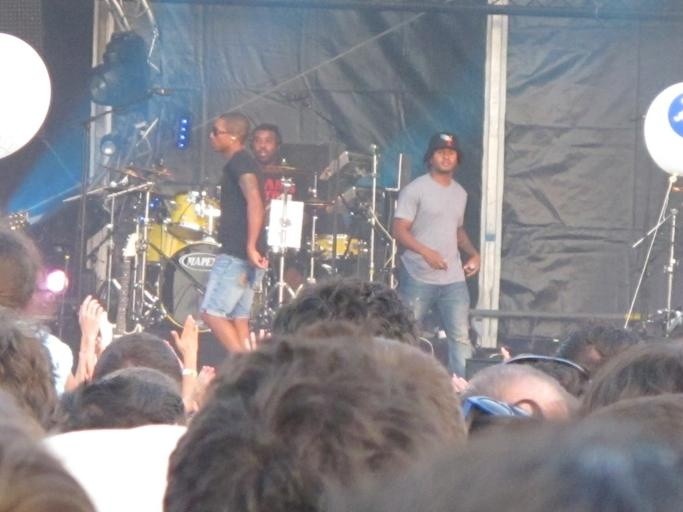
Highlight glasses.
[463,395,529,421]
[507,353,589,380]
[418,336,434,357]
[211,126,228,137]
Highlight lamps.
[86,33,147,105]
[101,135,120,155]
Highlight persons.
[203,114,267,361]
[164,333,469,512]
[390,134,480,376]
[251,126,301,299]
[244,282,417,351]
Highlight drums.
[317,233,366,260]
[157,242,221,332]
[164,189,220,245]
[140,222,189,267]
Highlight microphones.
[152,86,173,96]
[362,137,378,155]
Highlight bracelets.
[180,369,198,378]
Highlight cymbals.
[141,167,174,178]
[104,184,175,200]
[304,198,335,208]
[167,222,204,240]
[99,164,147,181]
[261,162,298,175]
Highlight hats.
[424,132,462,161]
